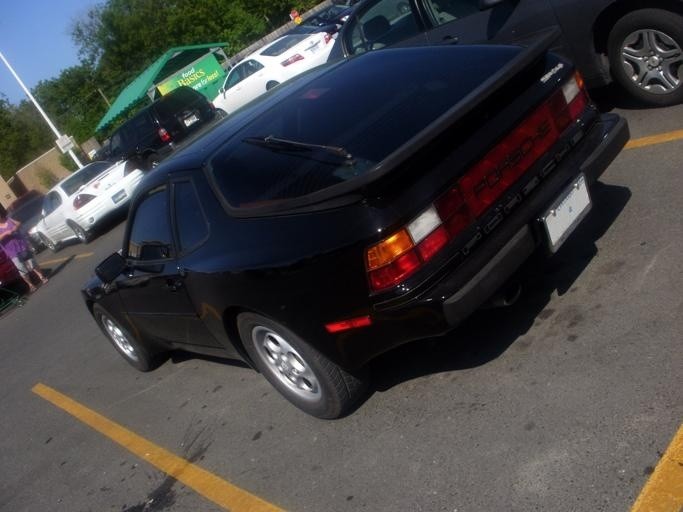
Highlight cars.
[80,46,629,422]
[0,161,142,305]
[210,0,351,121]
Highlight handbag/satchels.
[17,250,34,263]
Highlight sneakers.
[29,276,49,292]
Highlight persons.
[0,204,48,293]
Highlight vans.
[327,0,680,109]
[105,87,214,167]
